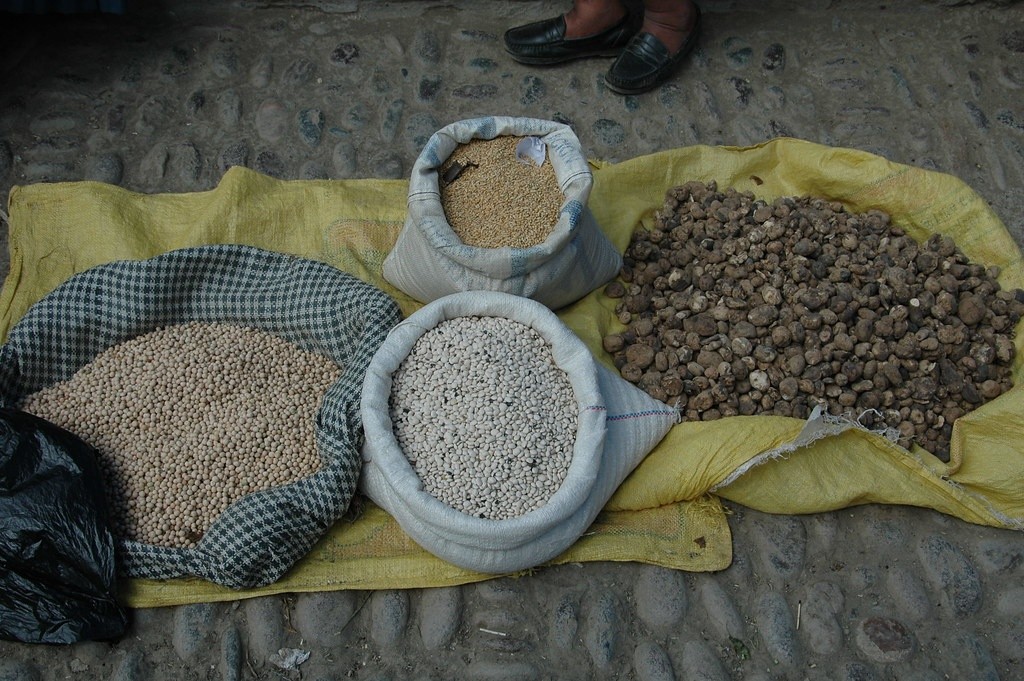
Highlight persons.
[504,0,702,95]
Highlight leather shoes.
[604,3,702,94]
[503,7,634,65]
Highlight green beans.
[9,321,342,549]
[389,316,577,520]
